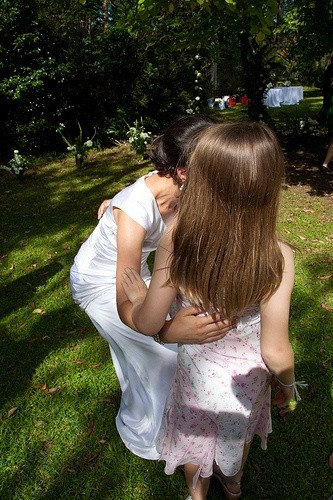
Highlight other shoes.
[320,164,333,174]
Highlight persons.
[121,122,296,500]
[321,55,333,172]
[69,115,215,460]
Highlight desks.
[263,86,303,108]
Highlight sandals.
[212,461,241,500]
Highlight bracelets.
[151,333,165,345]
[276,378,308,390]
